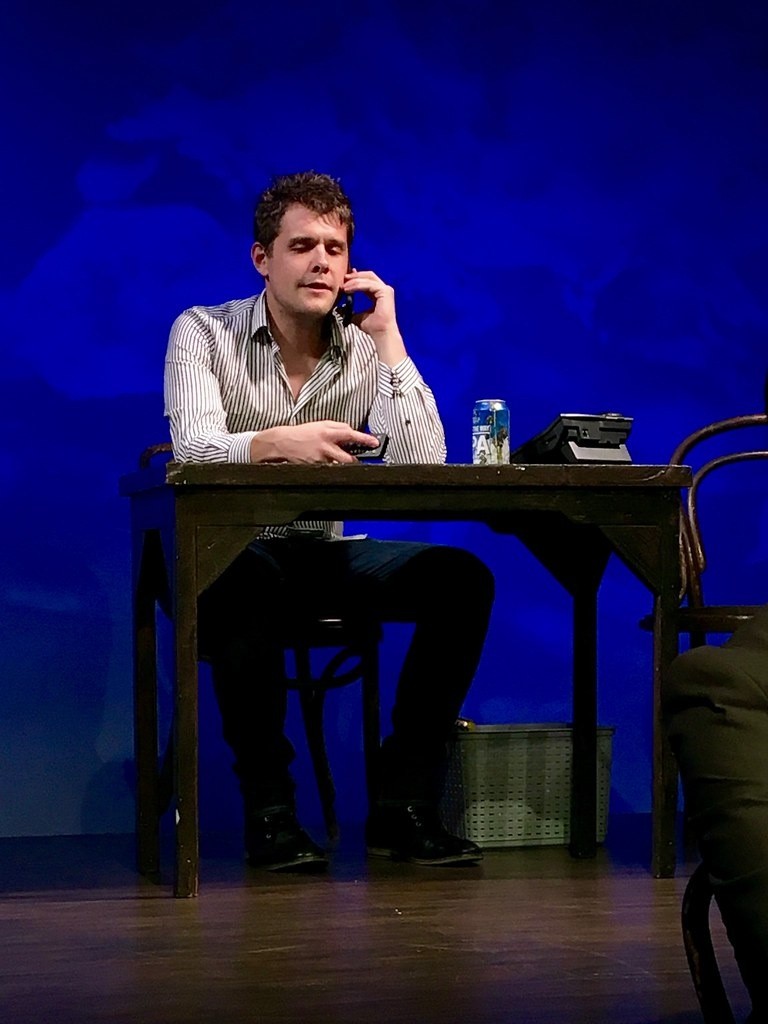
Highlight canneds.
[472,399,510,465]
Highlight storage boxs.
[438,722,614,850]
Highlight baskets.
[445,724,615,848]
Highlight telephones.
[510,410,634,466]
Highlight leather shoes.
[365,804,484,866]
[244,805,328,870]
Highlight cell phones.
[343,293,355,327]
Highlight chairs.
[635,413,768,880]
[140,442,389,867]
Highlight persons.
[164,173,495,875]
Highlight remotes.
[340,434,389,460]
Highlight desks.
[122,463,695,900]
